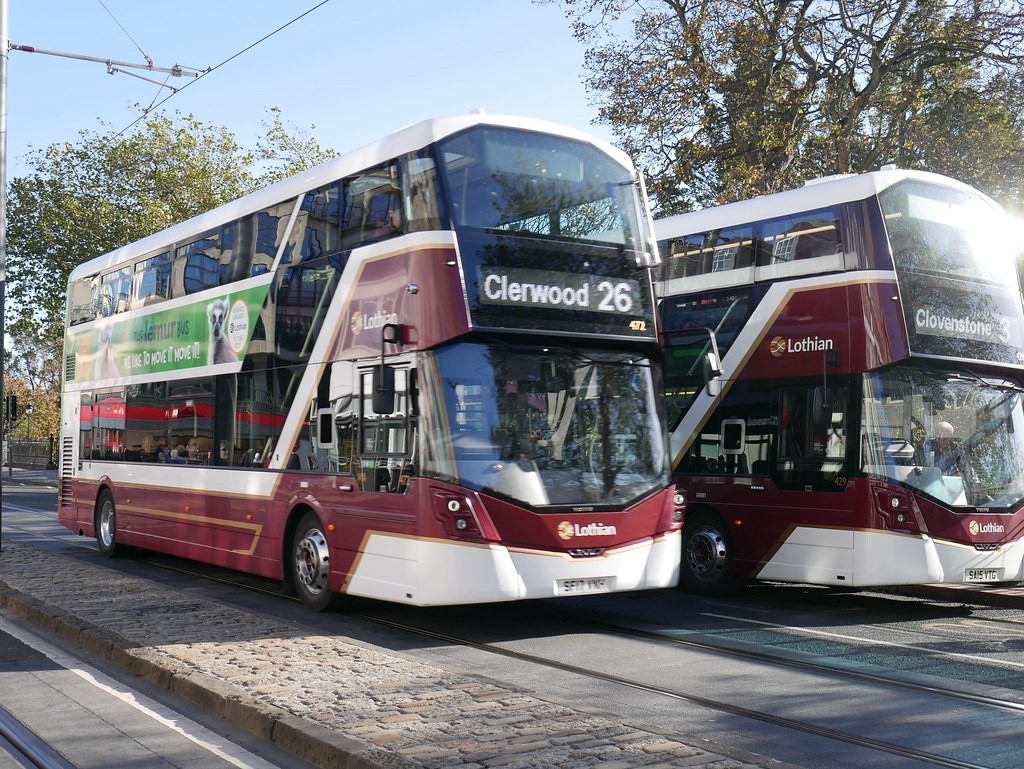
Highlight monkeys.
[207,295,239,365]
[95,324,119,379]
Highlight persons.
[373,195,401,237]
[140,434,242,467]
[914,423,981,493]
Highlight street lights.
[25,402,33,444]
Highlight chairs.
[675,455,770,475]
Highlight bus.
[653,164,1024,602]
[56,106,723,612]
